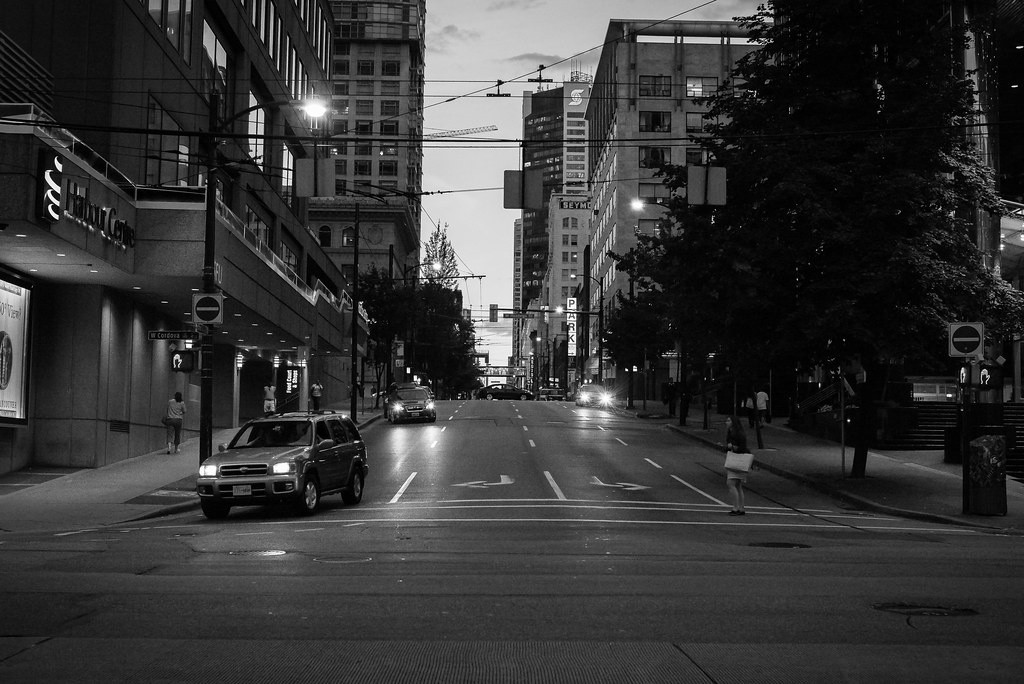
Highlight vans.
[540,388,564,401]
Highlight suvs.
[196,409,369,520]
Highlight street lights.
[403,261,441,286]
[570,274,604,385]
[199,98,328,464]
[536,337,556,388]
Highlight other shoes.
[176,448,180,453]
[728,510,746,516]
[167,448,172,454]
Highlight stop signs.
[192,292,224,325]
[947,322,983,357]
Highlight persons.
[310,378,323,411]
[286,423,325,446]
[744,387,770,429]
[166,392,186,453]
[725,414,751,516]
[245,427,274,446]
[264,379,277,415]
[665,377,678,416]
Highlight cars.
[380,383,435,419]
[387,388,436,425]
[575,384,611,408]
[477,384,535,401]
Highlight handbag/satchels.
[724,447,755,473]
[161,415,168,425]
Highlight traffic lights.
[979,364,1004,388]
[958,364,970,386]
[171,350,194,373]
